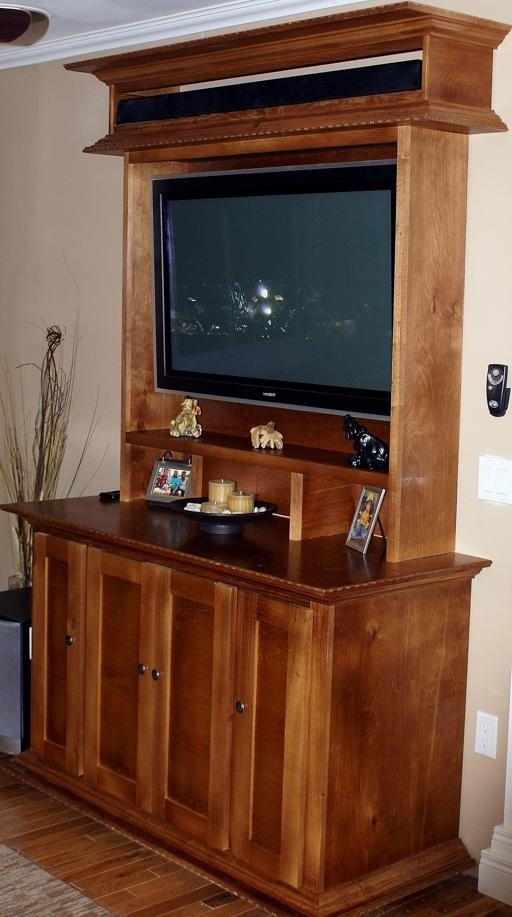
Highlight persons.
[354,499,375,533]
[153,468,172,496]
[177,471,191,497]
[169,470,180,496]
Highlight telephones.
[486,364,511,417]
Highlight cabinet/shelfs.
[119,125,469,562]
[238,577,478,917]
[89,544,233,881]
[28,528,88,778]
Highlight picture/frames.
[345,483,386,556]
[144,458,192,503]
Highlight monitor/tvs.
[148,158,398,423]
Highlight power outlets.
[475,711,500,761]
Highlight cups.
[199,479,255,514]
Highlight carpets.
[1,841,117,917]
